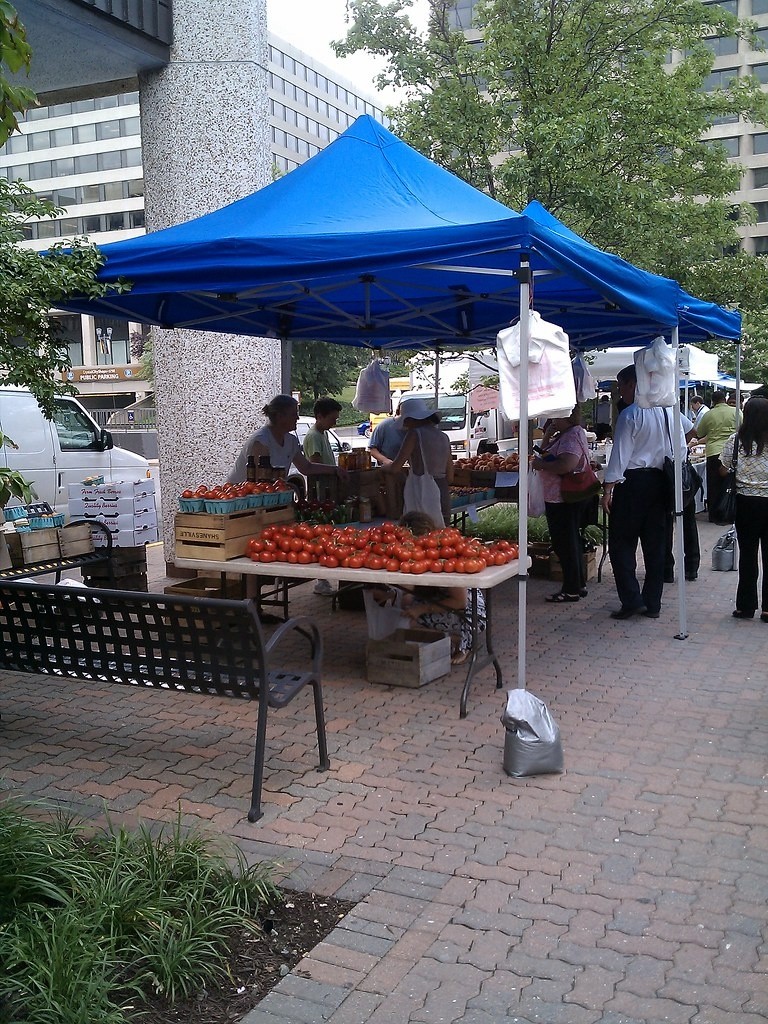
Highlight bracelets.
[429,604,434,614]
[603,489,612,494]
[335,466,339,475]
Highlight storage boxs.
[278,490,294,503]
[263,502,293,528]
[82,474,105,485]
[58,522,96,559]
[71,511,160,531]
[448,430,606,509]
[549,549,599,581]
[205,499,235,513]
[53,516,62,527]
[236,497,252,510]
[178,498,203,512]
[4,507,27,520]
[59,513,66,524]
[363,627,452,685]
[84,573,148,605]
[29,517,54,529]
[68,476,157,499]
[0,531,13,571]
[249,494,263,508]
[8,527,58,565]
[262,493,281,506]
[80,544,150,577]
[68,494,156,516]
[173,506,262,561]
[164,576,241,640]
[93,526,159,548]
[528,541,551,575]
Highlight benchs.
[0,499,113,584]
[0,568,332,822]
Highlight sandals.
[545,592,580,602]
[579,587,588,596]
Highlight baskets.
[81,476,104,486]
[3,506,65,532]
[452,490,495,508]
[177,490,294,514]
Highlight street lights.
[96,326,112,365]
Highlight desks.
[449,497,499,534]
[335,517,400,529]
[172,554,532,718]
[497,494,609,583]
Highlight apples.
[453,453,535,472]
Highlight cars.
[358,421,372,437]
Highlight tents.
[34,113,762,690]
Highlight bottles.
[338,447,372,471]
[245,453,286,483]
[359,496,372,523]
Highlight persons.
[615,398,700,583]
[602,363,687,619]
[597,394,612,438]
[368,401,409,466]
[679,384,768,525]
[538,400,591,602]
[303,397,342,596]
[383,398,454,529]
[367,511,487,665]
[224,394,351,623]
[719,395,768,623]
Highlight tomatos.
[181,479,288,500]
[247,522,519,574]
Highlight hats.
[397,399,443,430]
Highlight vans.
[0,368,150,514]
[286,416,353,500]
[369,377,410,435]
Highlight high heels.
[761,614,768,622]
[732,609,755,618]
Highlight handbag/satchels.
[527,459,546,519]
[361,587,401,640]
[708,432,739,526]
[663,456,704,509]
[402,428,446,530]
[556,433,602,504]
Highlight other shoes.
[685,569,697,578]
[611,603,648,619]
[664,575,674,583]
[644,606,660,617]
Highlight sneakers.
[313,580,338,595]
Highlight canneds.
[338,450,371,470]
[359,500,372,523]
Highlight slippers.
[450,642,483,665]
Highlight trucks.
[398,352,513,463]
